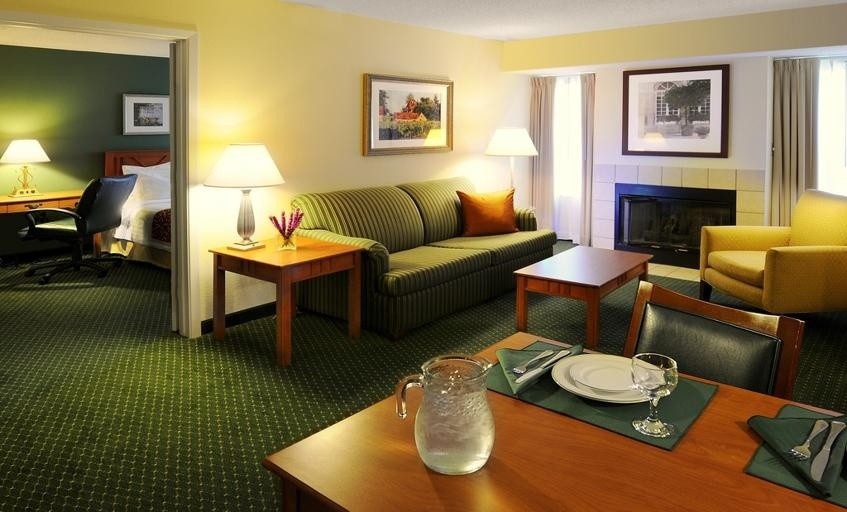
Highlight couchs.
[290,177,558,343]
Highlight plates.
[551,352,664,405]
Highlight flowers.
[268,209,304,247]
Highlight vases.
[279,233,296,250]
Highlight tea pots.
[391,357,501,478]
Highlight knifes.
[811,415,847,480]
[509,351,572,384]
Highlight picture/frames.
[122,93,170,135]
[363,71,454,155]
[621,63,733,159]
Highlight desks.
[207,233,366,369]
[0,189,102,258]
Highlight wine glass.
[631,353,680,436]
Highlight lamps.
[484,126,539,206]
[203,141,286,252]
[0,139,51,197]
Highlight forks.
[512,349,553,373]
[785,417,829,461]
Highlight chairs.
[624,281,806,399]
[698,189,847,337]
[16,173,139,285]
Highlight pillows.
[455,187,519,236]
[121,162,171,201]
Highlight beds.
[103,148,171,269]
